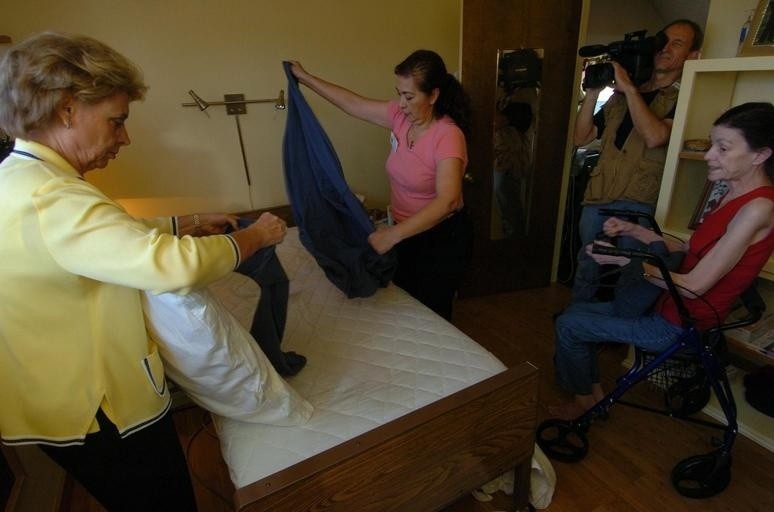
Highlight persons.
[290,48,474,327]
[572,17,705,306]
[0,30,288,512]
[553,102,773,417]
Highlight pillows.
[140,285,314,428]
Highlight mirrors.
[488,47,545,240]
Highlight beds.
[210,204,540,511]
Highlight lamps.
[181,89,287,120]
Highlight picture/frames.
[739,0,774,57]
[688,178,729,229]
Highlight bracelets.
[193,213,202,236]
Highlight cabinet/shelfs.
[619,55,774,455]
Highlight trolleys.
[535,208,767,498]
